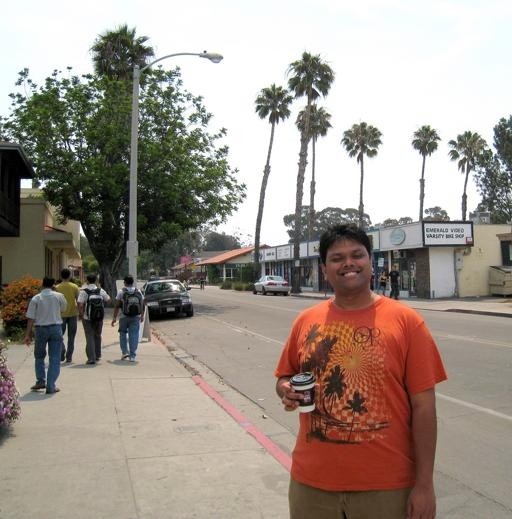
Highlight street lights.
[126,51,224,293]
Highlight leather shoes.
[30,381,45,389]
[46,388,59,394]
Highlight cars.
[141,280,193,319]
[252,275,291,298]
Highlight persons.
[274,225,446,519]
[54,269,81,364]
[76,275,110,366]
[111,275,146,362]
[388,265,399,300]
[200,274,205,290]
[25,276,67,395]
[378,271,387,296]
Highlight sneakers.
[61,348,102,364]
[129,357,134,362]
[121,353,130,360]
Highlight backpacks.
[122,287,143,316]
[83,286,104,322]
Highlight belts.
[35,324,59,327]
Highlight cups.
[291,373,317,414]
